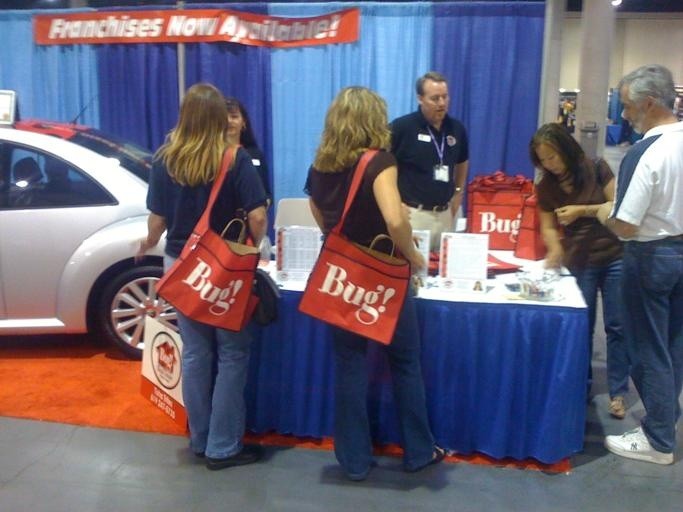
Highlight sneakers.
[604,426,673,466]
[196,446,257,470]
[611,396,626,417]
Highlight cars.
[1,125,175,356]
[1,117,156,182]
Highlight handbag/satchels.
[300,232,411,344]
[155,219,262,332]
[468,172,547,260]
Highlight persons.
[303,87,447,481]
[133,84,271,470]
[223,95,271,212]
[528,120,628,421]
[596,65,682,464]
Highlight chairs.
[273,196,320,247]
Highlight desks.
[239,259,591,466]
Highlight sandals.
[428,444,446,464]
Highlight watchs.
[390,72,471,252]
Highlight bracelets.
[453,186,464,192]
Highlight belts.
[401,197,450,211]
